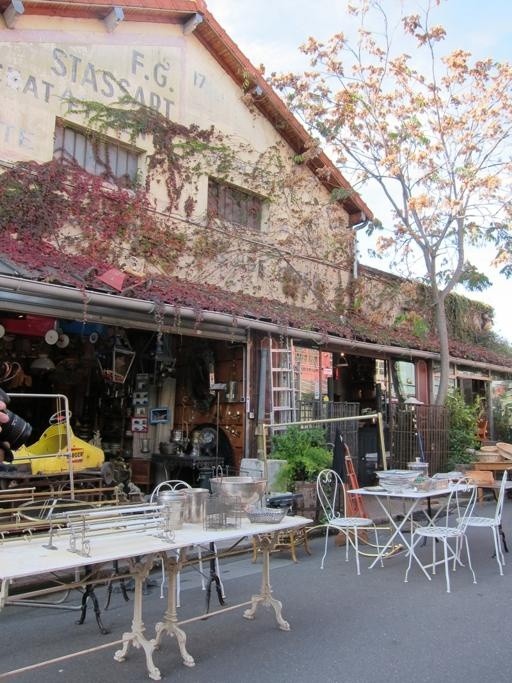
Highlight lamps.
[94,268,128,293]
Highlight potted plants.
[267,424,335,526]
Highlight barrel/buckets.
[209,465,267,514]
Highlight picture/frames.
[130,379,171,433]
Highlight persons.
[0,398,12,466]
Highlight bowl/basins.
[210,475,269,518]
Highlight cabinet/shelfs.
[94,393,133,456]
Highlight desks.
[149,453,225,495]
[127,458,150,494]
[469,460,512,502]
[0,514,314,682]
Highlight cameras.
[0,387,32,452]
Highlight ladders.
[326,443,368,517]
[268,335,296,454]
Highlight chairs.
[317,470,384,575]
[453,471,508,577]
[250,528,312,564]
[403,475,478,594]
[464,471,512,507]
[150,480,226,607]
